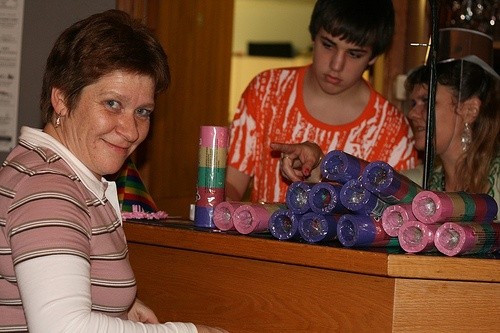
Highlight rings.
[283,154,293,163]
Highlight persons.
[221,0,419,205]
[0,9,229,333]
[267,58,500,226]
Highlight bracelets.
[302,138,324,170]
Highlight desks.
[122,217,500,333]
